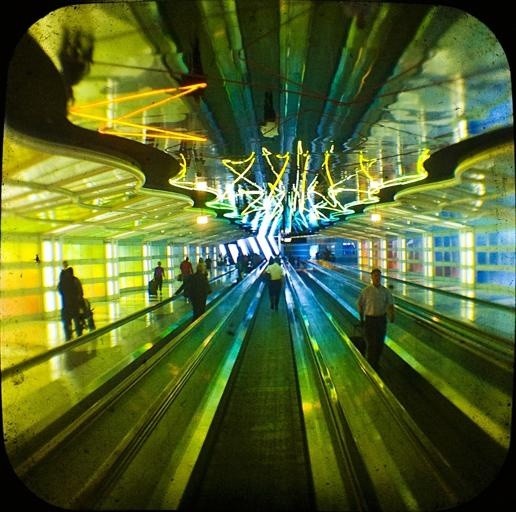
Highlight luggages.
[349,325,366,358]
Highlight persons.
[191,263,212,321]
[315,248,335,261]
[217,254,222,266]
[205,256,212,269]
[199,258,208,280]
[288,256,306,269]
[237,252,261,280]
[57,267,84,342]
[154,262,165,296]
[261,257,283,312]
[174,257,193,296]
[224,257,227,264]
[356,268,394,368]
